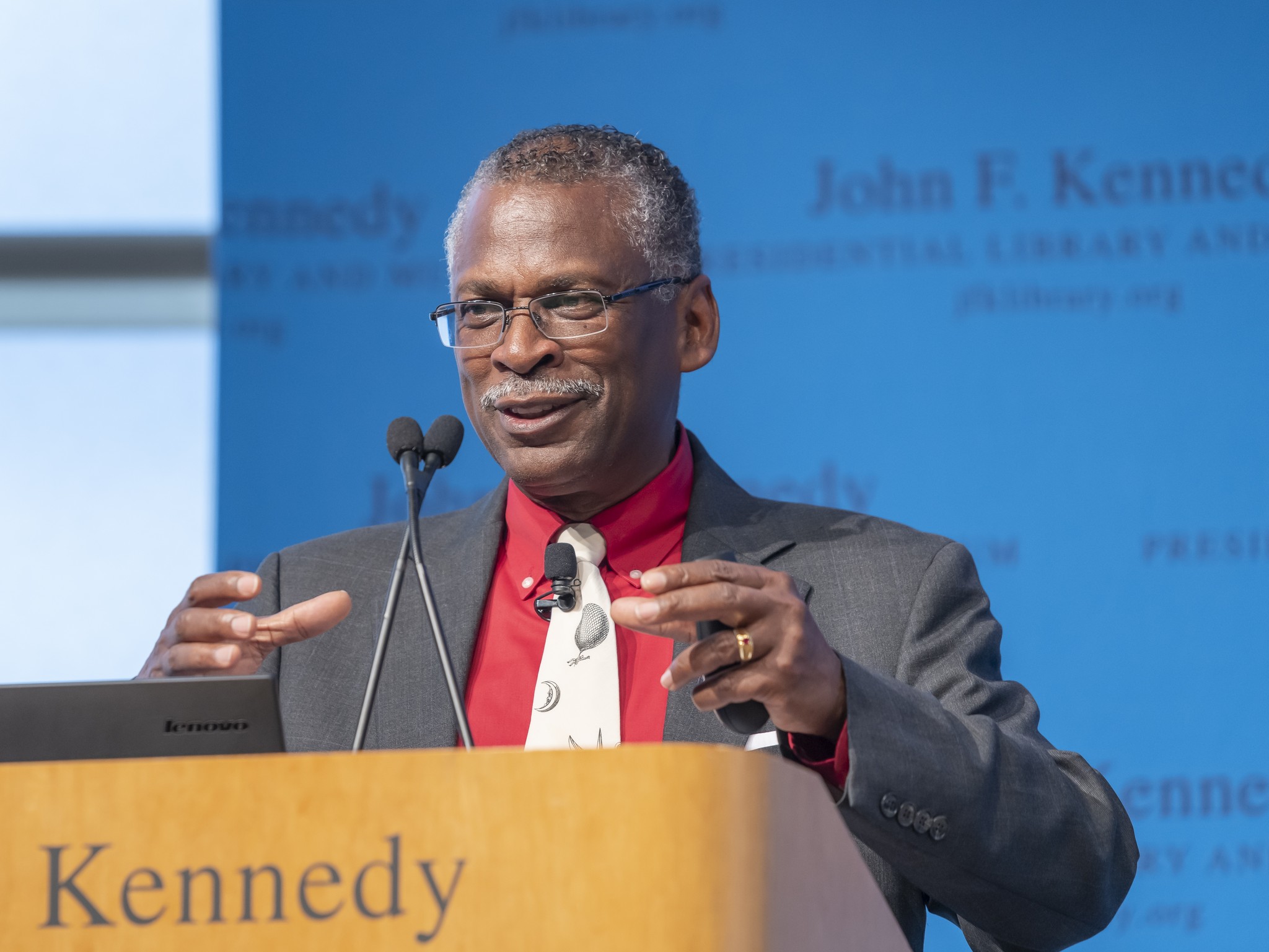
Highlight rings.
[734,626,754,664]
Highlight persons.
[130,123,1141,952]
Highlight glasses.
[429,271,698,348]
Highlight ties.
[524,521,622,754]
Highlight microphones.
[354,413,476,750]
[533,542,577,612]
